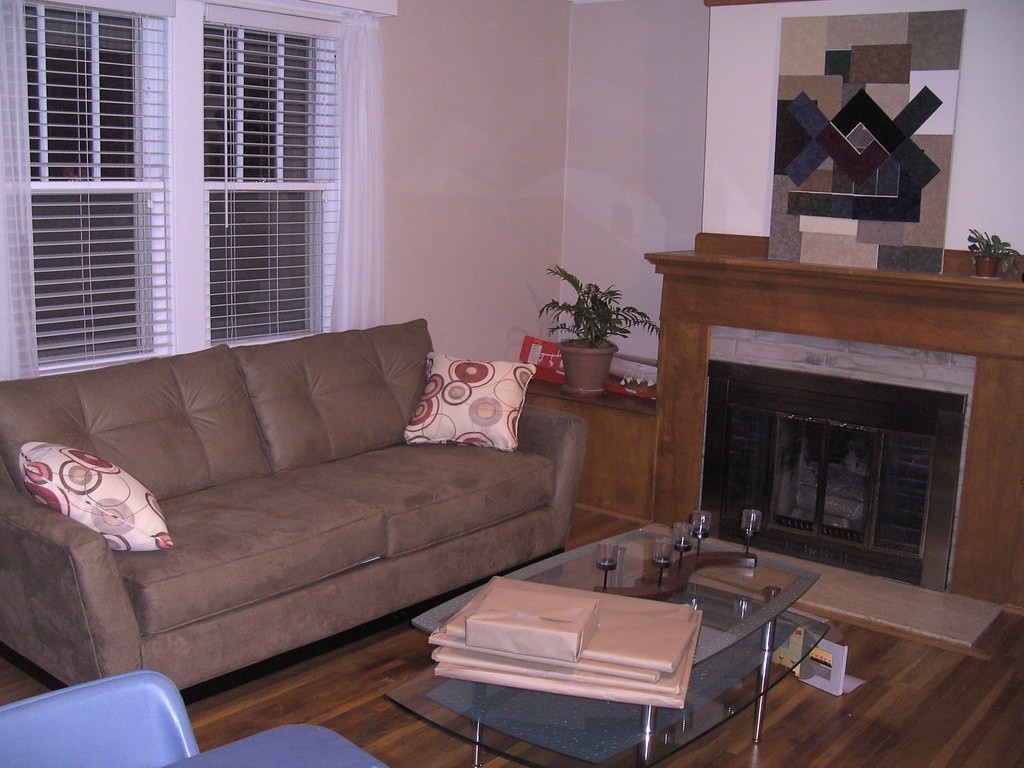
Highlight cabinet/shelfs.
[521,376,659,529]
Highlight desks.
[163,725,391,768]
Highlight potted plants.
[967,229,1020,278]
[539,264,661,395]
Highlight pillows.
[403,352,537,453]
[18,441,174,552]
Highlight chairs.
[0,670,201,768]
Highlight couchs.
[0,318,590,694]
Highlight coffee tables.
[385,524,831,768]
